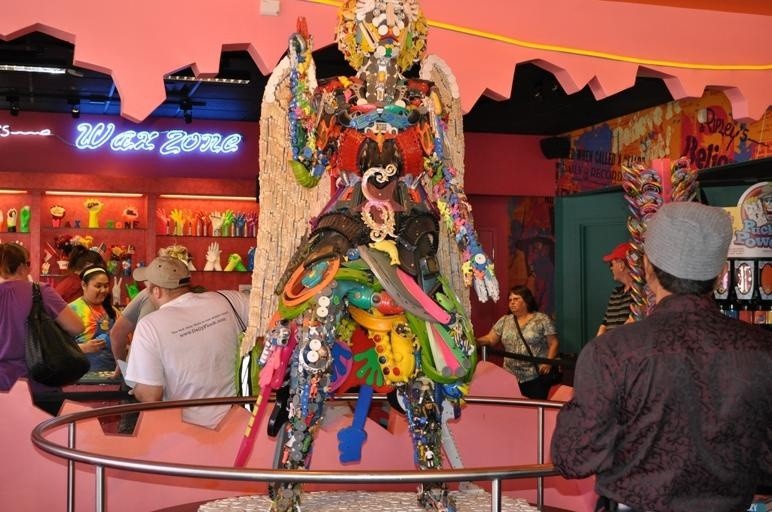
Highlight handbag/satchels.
[236,332,263,413]
[23,281,90,387]
[542,364,562,384]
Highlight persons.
[122,253,251,430]
[0,241,86,397]
[107,286,158,376]
[593,242,639,336]
[65,264,123,370]
[468,283,564,400]
[547,201,771,512]
[236,0,504,511]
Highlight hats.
[132,255,191,289]
[605,243,635,262]
[645,202,733,282]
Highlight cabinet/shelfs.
[0,191,31,278]
[155,198,259,274]
[39,191,149,308]
